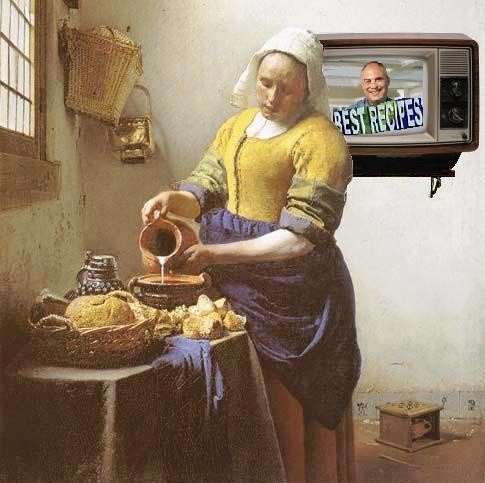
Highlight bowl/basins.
[127,273,213,312]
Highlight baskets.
[105,83,154,164]
[28,289,160,363]
[63,26,144,127]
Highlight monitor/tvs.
[314,44,474,149]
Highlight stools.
[375,400,446,454]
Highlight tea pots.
[139,209,197,273]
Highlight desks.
[13,327,252,481]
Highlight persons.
[140,27,362,481]
[345,60,402,133]
